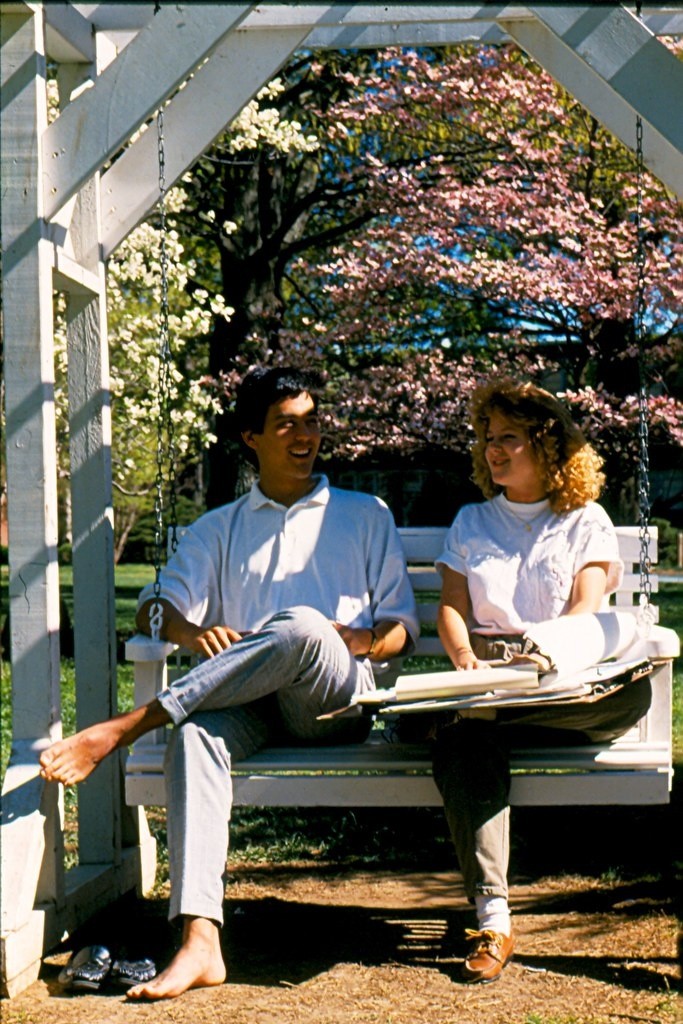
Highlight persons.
[39,373,421,998]
[430,382,651,984]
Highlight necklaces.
[504,500,552,531]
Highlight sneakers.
[462,918,513,984]
[396,709,440,743]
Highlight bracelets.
[363,629,377,658]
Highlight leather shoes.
[57,924,117,991]
[112,929,162,985]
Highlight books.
[395,664,540,700]
